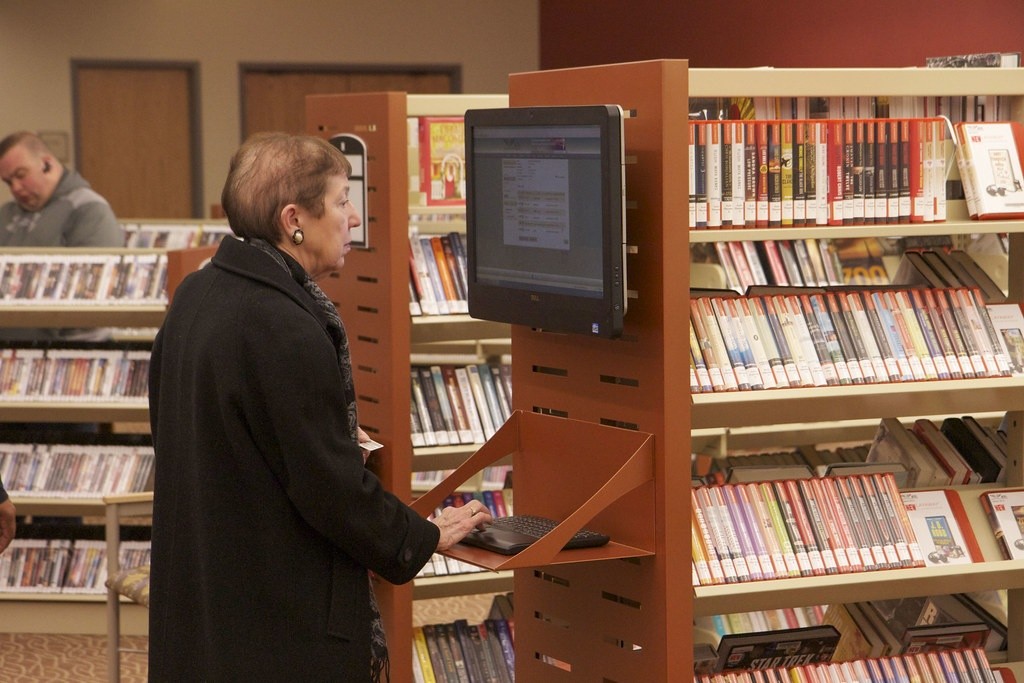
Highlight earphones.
[43,162,50,172]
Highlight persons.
[147,131,496,683]
[0,478,17,554]
[0,131,125,541]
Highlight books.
[405,115,469,318]
[691,415,1024,588]
[0,443,155,500]
[411,591,515,683]
[411,487,513,579]
[119,222,233,253]
[0,539,150,595]
[693,594,1007,683]
[0,348,151,402]
[688,243,1024,393]
[1,253,169,304]
[689,54,1024,231]
[409,355,513,448]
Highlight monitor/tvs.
[463,105,630,340]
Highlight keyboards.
[465,513,610,555]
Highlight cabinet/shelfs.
[0,57,1024,682]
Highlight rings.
[470,506,475,515]
